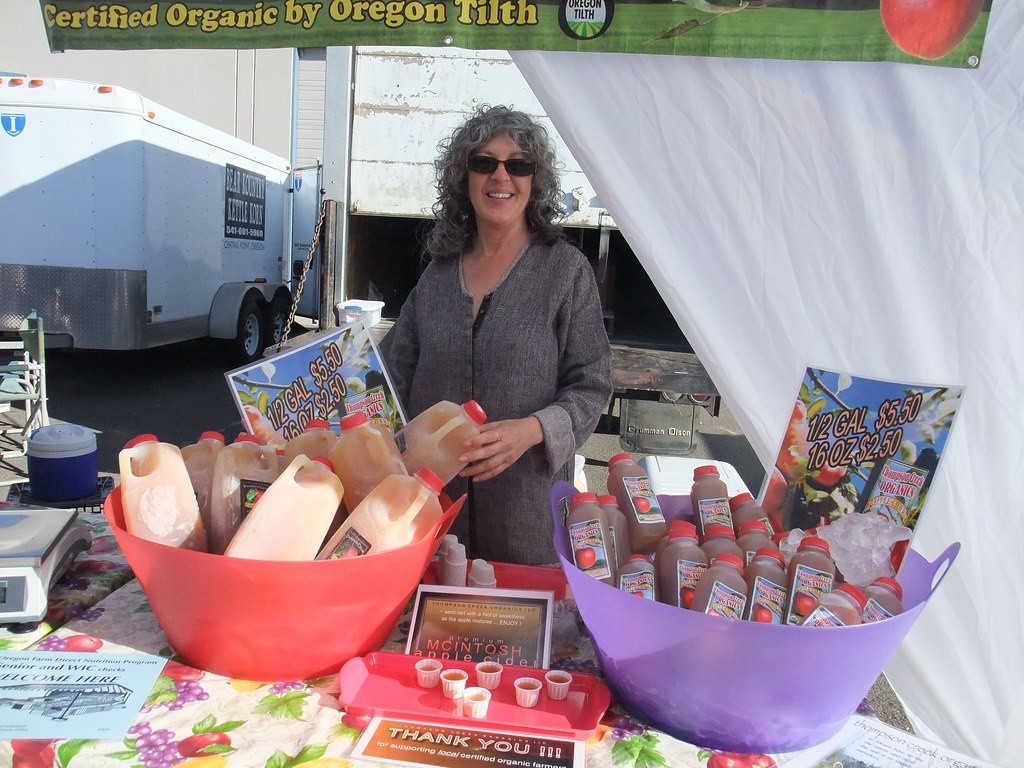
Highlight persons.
[378,106,612,566]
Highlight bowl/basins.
[104,484,467,682]
[549,481,961,755]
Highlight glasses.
[467,155,534,176]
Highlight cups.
[513,677,542,708]
[475,661,503,690]
[545,670,572,701]
[415,658,443,688]
[439,669,468,700]
[462,687,492,718]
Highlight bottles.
[118,400,487,561]
[437,534,468,587]
[567,452,905,638]
[467,559,497,589]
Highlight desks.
[0,499,888,768]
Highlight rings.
[497,433,498,437]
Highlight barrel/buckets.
[25,423,100,502]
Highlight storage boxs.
[336,299,386,329]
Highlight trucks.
[0,70,322,368]
